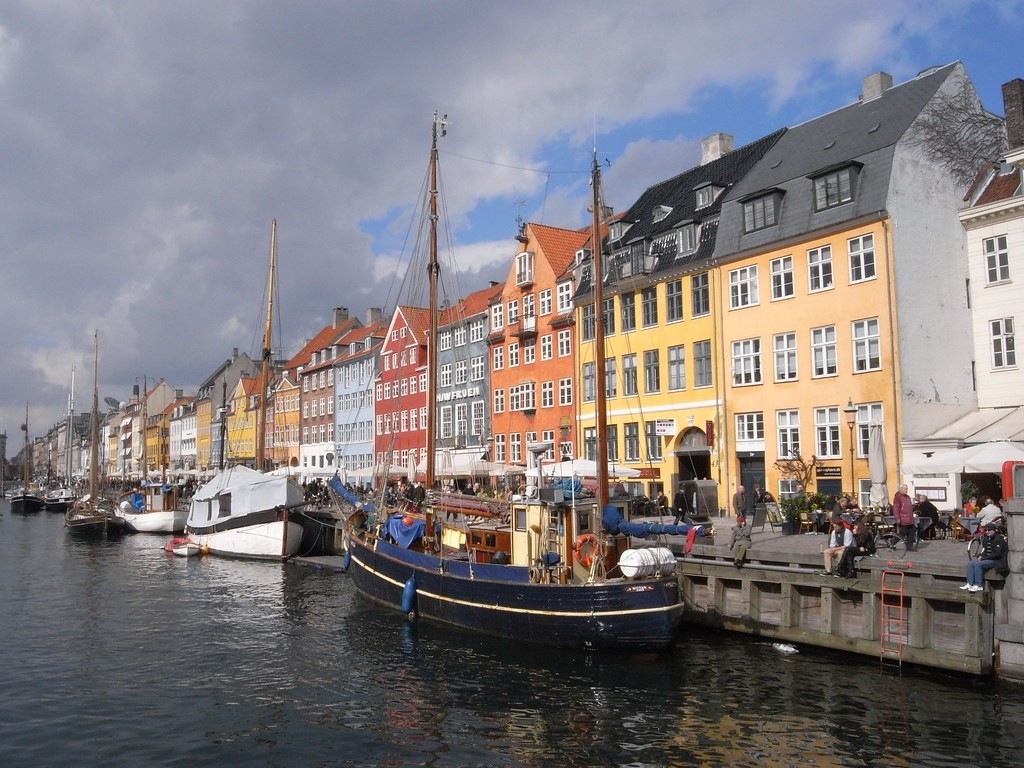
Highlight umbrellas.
[107,468,221,477]
[456,459,527,475]
[525,457,641,478]
[869,420,889,512]
[902,441,1024,474]
[442,446,455,485]
[268,465,408,476]
[408,449,417,482]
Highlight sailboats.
[114,374,189,534]
[183,217,320,562]
[325,108,689,645]
[10,399,42,513]
[64,328,127,535]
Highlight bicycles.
[864,513,908,560]
[967,521,1006,562]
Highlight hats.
[984,524,996,531]
[736,517,745,522]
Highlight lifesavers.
[574,535,598,567]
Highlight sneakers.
[959,583,972,590]
[968,585,983,592]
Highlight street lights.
[843,396,860,500]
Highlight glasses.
[984,529,991,531]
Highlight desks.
[957,517,981,536]
[884,517,933,534]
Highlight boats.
[164,536,201,558]
[4,482,74,513]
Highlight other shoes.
[734,559,740,565]
[834,573,841,577]
[887,544,896,550]
[820,572,832,577]
[906,547,917,551]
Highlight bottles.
[964,504,968,517]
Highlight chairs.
[799,502,982,545]
[639,498,668,516]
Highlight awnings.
[418,451,486,475]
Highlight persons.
[796,485,802,497]
[655,490,666,506]
[673,485,688,525]
[387,481,425,507]
[728,516,752,568]
[914,494,939,530]
[888,484,918,552]
[733,486,746,517]
[111,478,123,489]
[966,497,977,514]
[820,497,876,578]
[750,484,761,516]
[959,523,1008,591]
[302,479,372,503]
[179,476,206,496]
[977,498,1001,526]
[447,482,526,520]
